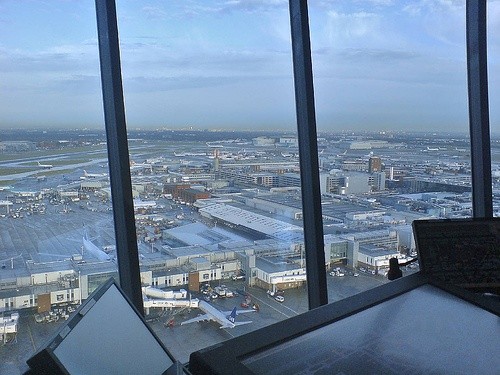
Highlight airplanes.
[1,200,14,206]
[83,169,108,177]
[173,142,299,161]
[180,299,257,330]
[37,162,53,170]
[133,196,157,210]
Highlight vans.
[34,305,75,323]
[267,288,285,302]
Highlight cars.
[329,266,359,277]
[201,282,239,301]
[361,266,376,275]
